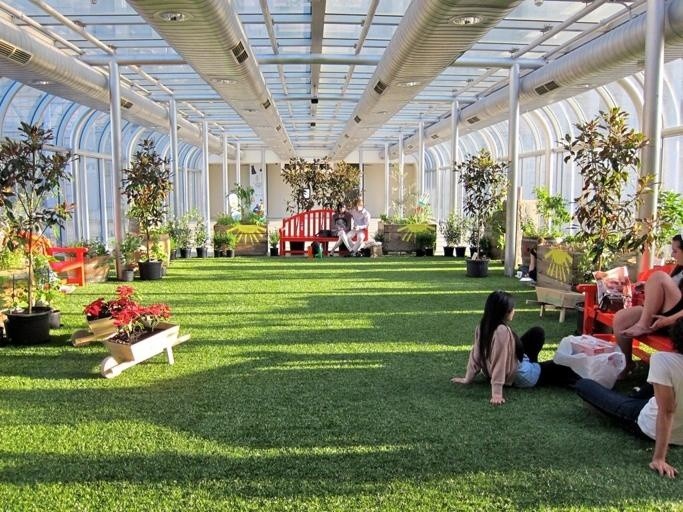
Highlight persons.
[450,290,581,409]
[575,316,683,479]
[329,201,355,257]
[343,199,371,258]
[611,234,683,379]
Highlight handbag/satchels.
[319,230,331,236]
[603,293,623,312]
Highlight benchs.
[576,262,683,369]
[278,208,369,257]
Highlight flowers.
[81,285,173,341]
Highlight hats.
[354,199,363,207]
[338,201,345,208]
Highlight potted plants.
[414,147,512,278]
[62,136,238,283]
[518,184,574,270]
[0,120,80,346]
[268,225,279,256]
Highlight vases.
[100,322,181,368]
